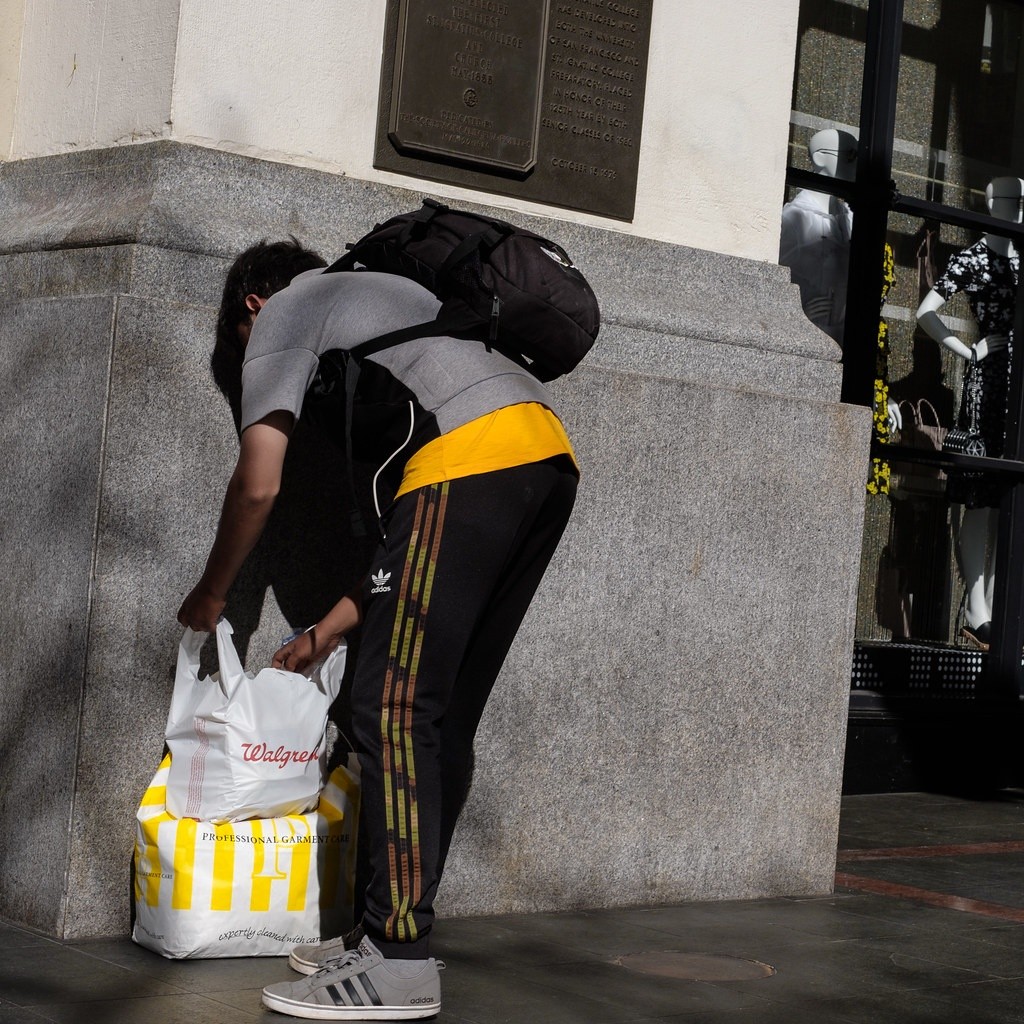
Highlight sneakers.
[288,919,364,977]
[262,934,446,1019]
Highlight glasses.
[817,147,857,163]
[994,194,1023,210]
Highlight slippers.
[962,619,992,649]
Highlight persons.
[916,177,1024,651]
[177,240,581,1020]
[782,127,892,494]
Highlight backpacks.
[316,197,601,384]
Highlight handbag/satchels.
[898,399,948,450]
[130,743,348,959]
[165,614,346,827]
